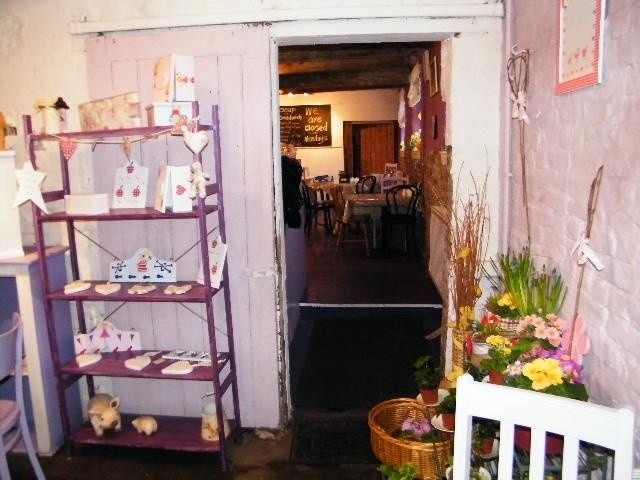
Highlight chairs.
[356,175,376,195]
[453,372,634,480]
[384,162,398,177]
[334,190,370,258]
[0,312,46,480]
[380,185,419,258]
[380,174,409,194]
[301,180,329,235]
[306,187,333,238]
[314,175,333,183]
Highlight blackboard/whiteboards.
[279,104,332,147]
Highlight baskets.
[367,398,455,475]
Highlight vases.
[452,329,473,371]
[485,309,519,341]
[514,424,562,455]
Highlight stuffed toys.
[89,394,121,436]
[133,413,158,436]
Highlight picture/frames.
[422,49,430,83]
[431,114,437,139]
[430,56,438,97]
[554,0,606,96]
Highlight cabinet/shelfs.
[0,244,77,457]
[23,101,243,474]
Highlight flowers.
[486,292,521,322]
[515,310,570,349]
[396,412,435,442]
[428,142,492,333]
[480,249,568,318]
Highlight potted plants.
[478,334,510,385]
[414,353,442,404]
[471,316,504,364]
[472,417,496,455]
[338,168,350,182]
[504,358,588,456]
[448,438,491,480]
[436,393,456,430]
